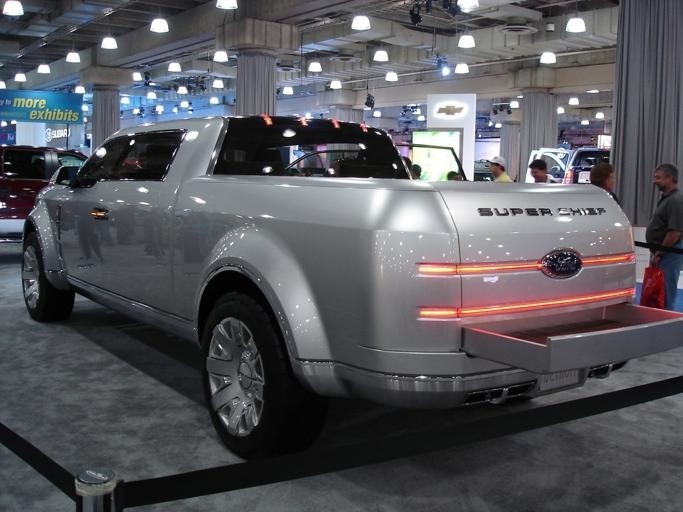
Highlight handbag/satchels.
[640,268,665,309]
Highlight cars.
[474,158,498,182]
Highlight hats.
[487,156,505,166]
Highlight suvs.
[523,144,611,184]
[0,144,142,243]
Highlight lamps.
[67,39,80,64]
[101,19,118,50]
[282,0,606,129]
[75,51,229,125]
[38,51,51,75]
[14,59,26,81]
[2,1,24,16]
[150,10,168,33]
[215,2,238,11]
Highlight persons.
[643,163,682,311]
[447,172,459,181]
[486,157,512,181]
[590,163,618,205]
[529,159,557,183]
[412,165,421,179]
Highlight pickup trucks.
[19,112,682,449]
